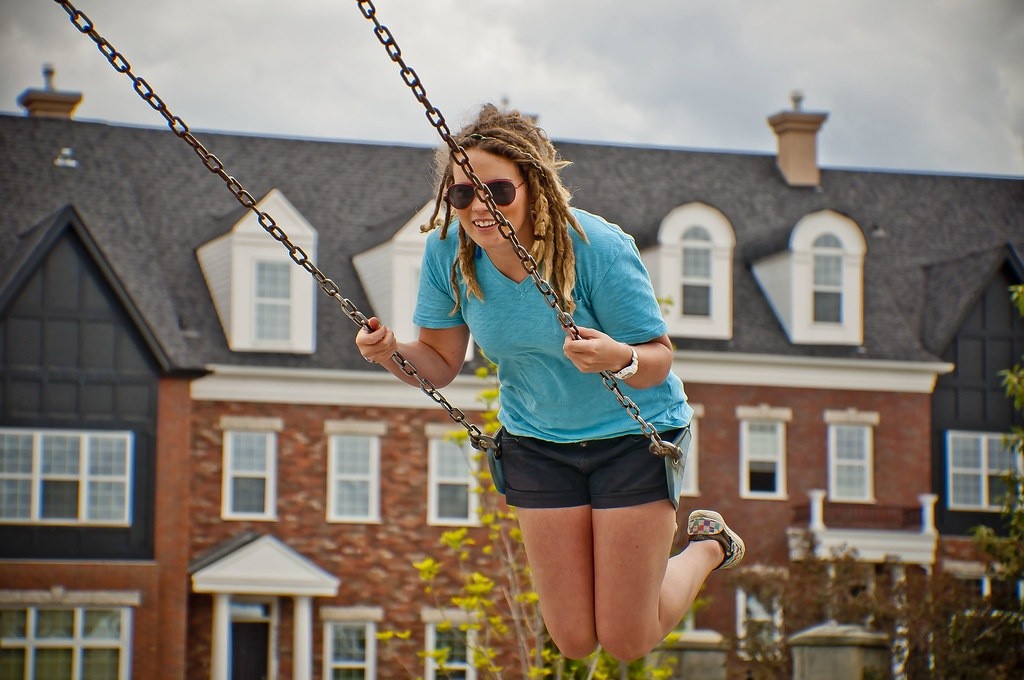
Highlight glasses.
[443,179,527,210]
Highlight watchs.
[612,346,638,380]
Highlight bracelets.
[364,357,379,364]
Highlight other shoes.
[686,511,745,572]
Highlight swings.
[51,1,693,510]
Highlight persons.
[356,102,744,662]
